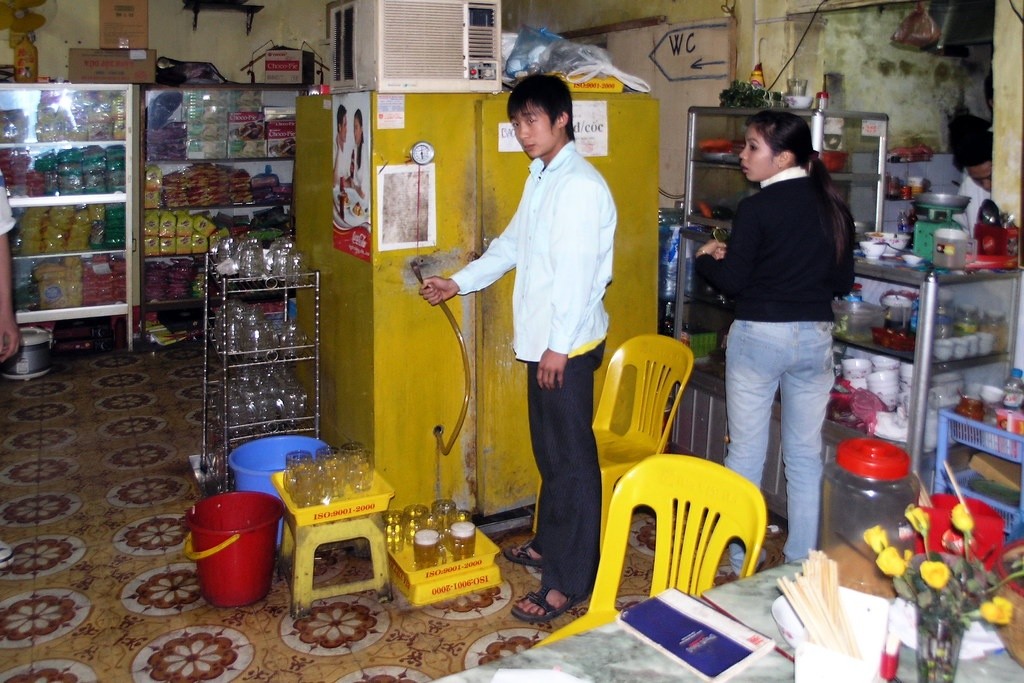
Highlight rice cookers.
[0,327,54,380]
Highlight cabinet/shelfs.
[672,107,1024,532]
[139,80,302,341]
[200,252,323,493]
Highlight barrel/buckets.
[185,434,328,608]
[823,150,850,172]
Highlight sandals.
[511,585,588,621]
[504,541,542,566]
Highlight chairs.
[532,333,692,554]
[532,452,769,649]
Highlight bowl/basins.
[841,355,1004,453]
[783,96,814,108]
[864,232,912,257]
[901,254,921,264]
[934,332,993,361]
[835,300,889,335]
[771,594,807,649]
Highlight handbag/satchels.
[505,25,650,92]
[891,2,942,48]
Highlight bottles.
[749,61,829,112]
[813,439,1006,605]
[843,282,862,300]
[898,209,918,248]
[935,304,1008,351]
[658,208,733,340]
[956,368,1024,451]
[888,176,923,199]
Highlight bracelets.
[696,249,707,254]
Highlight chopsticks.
[778,549,858,659]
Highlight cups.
[283,441,370,508]
[786,77,808,96]
[882,294,912,331]
[933,229,977,269]
[214,236,302,281]
[384,500,476,570]
[213,300,308,436]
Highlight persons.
[693,113,856,574]
[0,170,25,572]
[416,75,614,625]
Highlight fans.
[0,0,57,35]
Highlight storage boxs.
[271,463,395,525]
[67,47,156,85]
[98,0,149,49]
[384,521,502,606]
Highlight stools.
[282,509,393,619]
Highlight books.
[613,586,777,683]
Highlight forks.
[859,241,887,258]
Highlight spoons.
[873,242,912,254]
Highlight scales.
[911,192,971,262]
